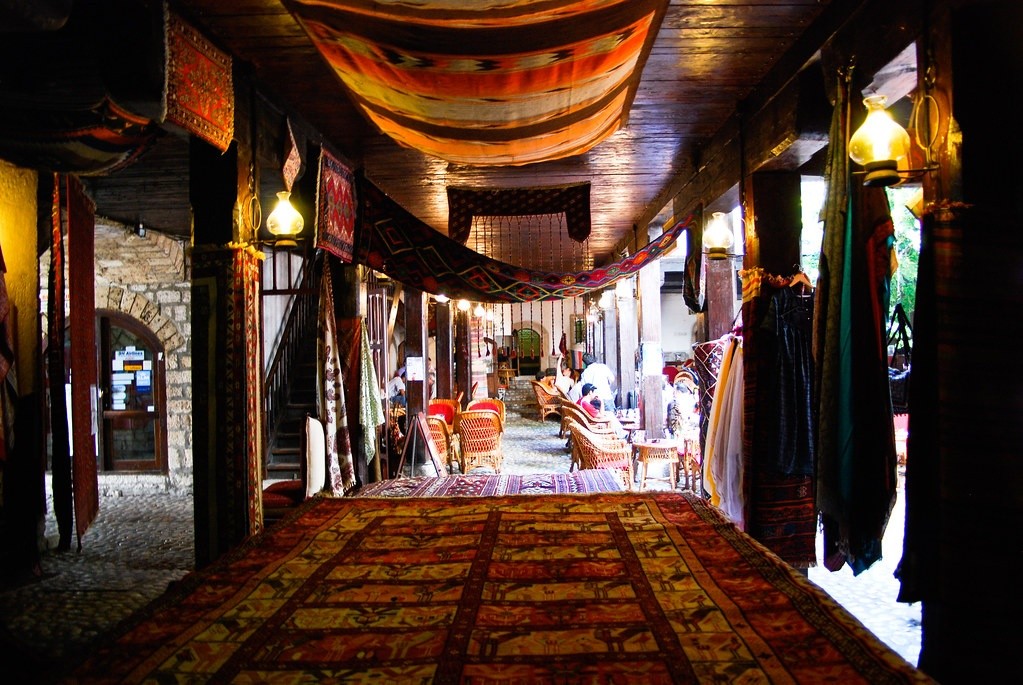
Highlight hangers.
[789,263,815,298]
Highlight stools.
[498,369,517,390]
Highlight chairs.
[391,382,505,474]
[663,358,695,389]
[675,428,702,492]
[532,367,634,489]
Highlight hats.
[583,353,594,365]
[582,384,597,394]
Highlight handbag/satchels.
[884,304,914,417]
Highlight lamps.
[245,189,305,247]
[847,96,939,187]
[702,212,748,260]
[586,302,603,322]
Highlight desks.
[618,418,643,443]
[633,438,678,493]
[665,361,684,367]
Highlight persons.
[580,353,617,411]
[577,383,629,436]
[399,360,406,376]
[387,372,406,407]
[555,353,579,394]
[536,372,562,397]
[428,357,433,385]
